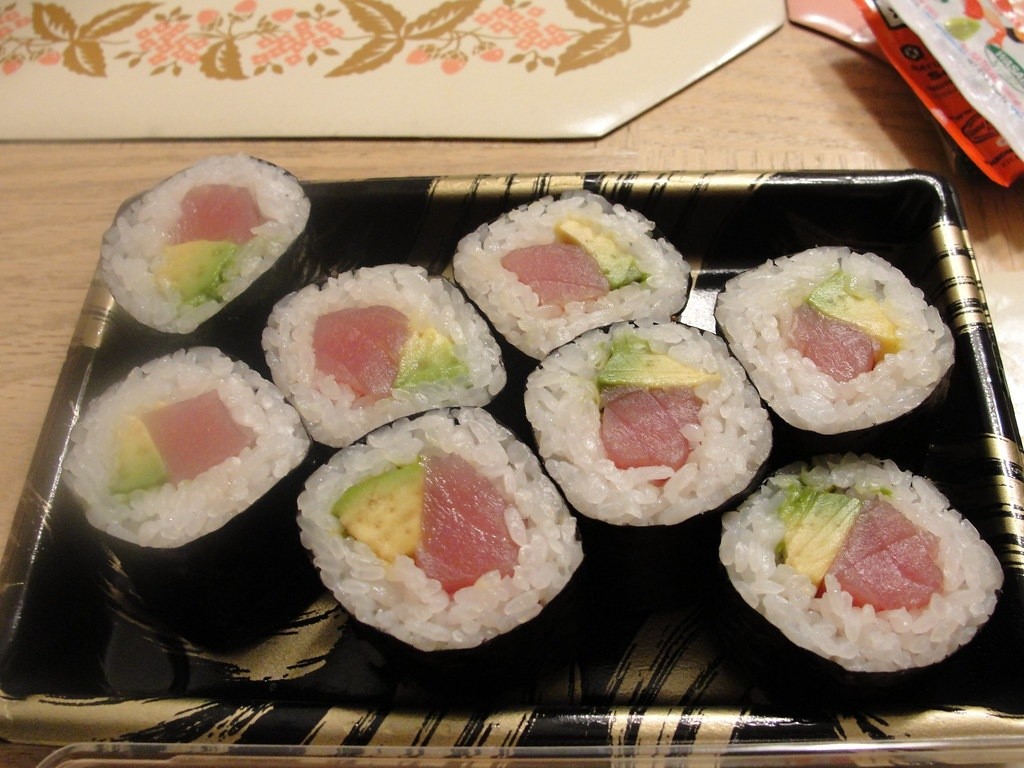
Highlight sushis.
[58,152,1002,715]
